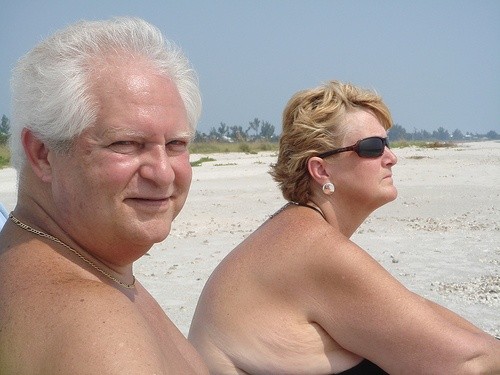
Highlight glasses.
[318,135,390,158]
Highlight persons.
[188,79,500,375]
[0,16,211,375]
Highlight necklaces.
[9,211,136,288]
[268,200,330,224]
